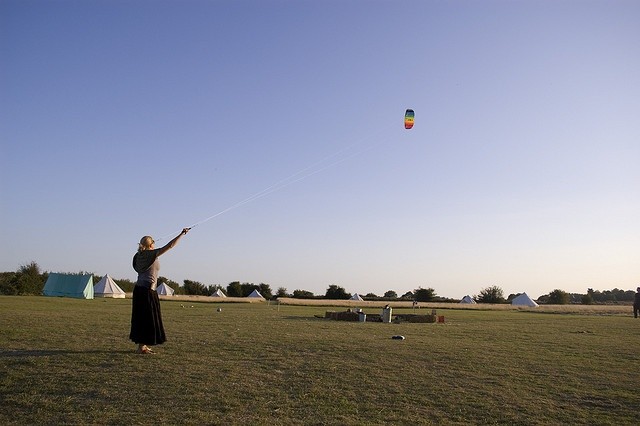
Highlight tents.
[511,291,539,307]
[95,274,126,298]
[42,272,95,299]
[459,296,476,303]
[349,293,364,301]
[156,282,174,296]
[248,289,263,297]
[211,288,226,297]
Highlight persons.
[130,227,191,354]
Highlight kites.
[404,109,414,129]
